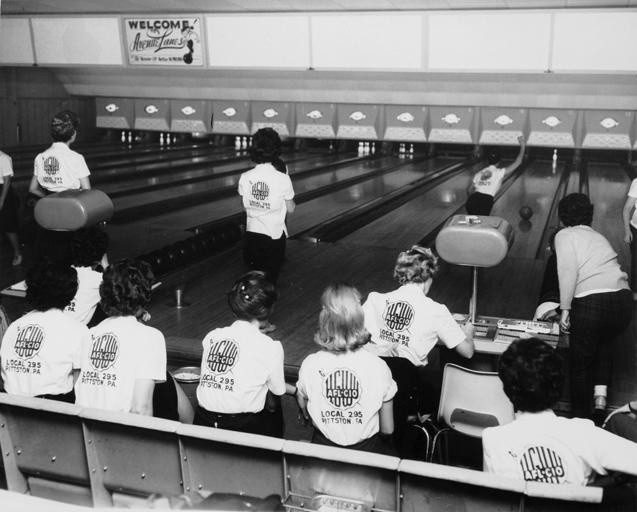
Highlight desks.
[452,313,560,360]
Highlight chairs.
[178,423,285,512]
[282,440,401,511]
[77,407,184,512]
[522,482,603,511]
[0,392,94,509]
[380,356,434,462]
[398,459,527,512]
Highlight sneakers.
[594,396,606,411]
[259,319,275,334]
[12,254,24,266]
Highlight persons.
[28,111,107,270]
[294,285,399,455]
[72,258,179,422]
[622,179,637,300]
[0,152,23,266]
[465,136,526,216]
[544,194,635,414]
[237,127,296,288]
[196,270,298,437]
[362,246,475,412]
[0,258,92,406]
[482,336,637,491]
